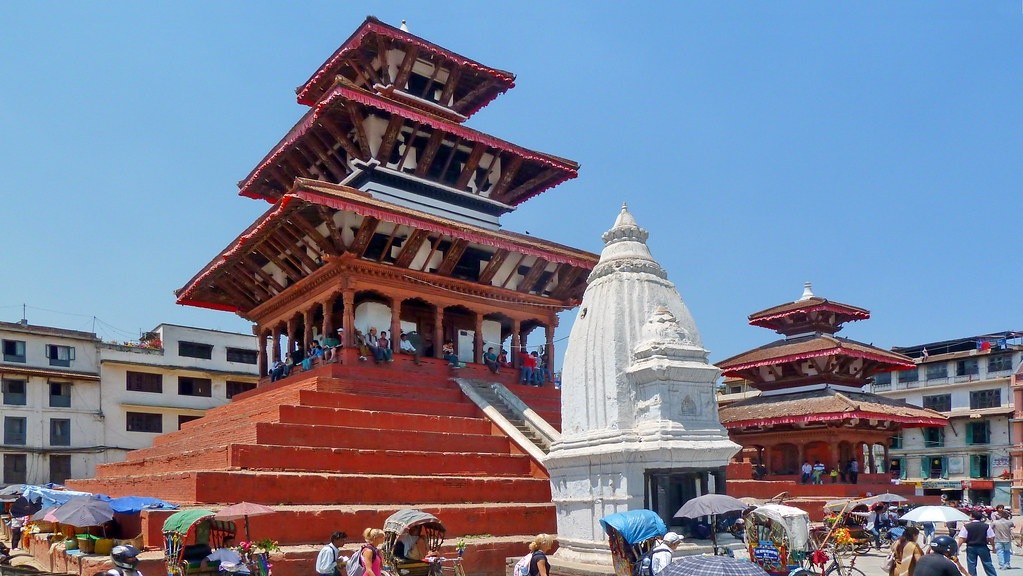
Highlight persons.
[800,456,859,486]
[316,530,348,576]
[651,531,684,575]
[201,535,250,573]
[10,516,24,551]
[848,496,1023,576]
[359,527,385,576]
[394,525,422,559]
[351,326,555,387]
[529,533,553,576]
[104,544,143,576]
[735,518,744,542]
[268,325,344,383]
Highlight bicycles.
[1008,524,1023,556]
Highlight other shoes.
[13,548,20,551]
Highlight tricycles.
[161,507,272,576]
[744,503,865,576]
[819,498,895,555]
[599,509,736,576]
[378,509,466,576]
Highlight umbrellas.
[29,497,114,527]
[214,501,277,540]
[655,555,771,576]
[673,493,785,555]
[898,506,972,522]
[870,493,907,520]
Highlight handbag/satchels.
[880,551,896,574]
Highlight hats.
[337,328,342,332]
[664,532,684,545]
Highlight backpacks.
[513,552,540,576]
[345,546,376,576]
[633,545,672,576]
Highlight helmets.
[110,545,140,569]
[930,536,959,559]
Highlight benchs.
[167,543,220,573]
[846,524,870,545]
